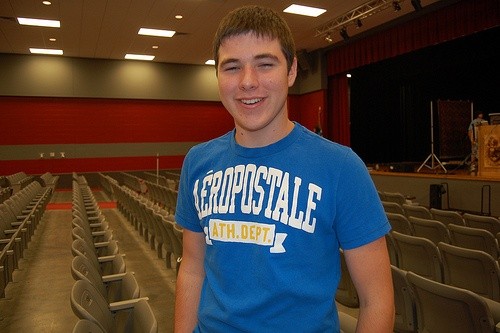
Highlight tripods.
[416,101,447,173]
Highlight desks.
[477,125,500,178]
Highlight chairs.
[0,171,500,333]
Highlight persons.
[468,111,489,177]
[174,6,394,333]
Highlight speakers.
[430,184,441,210]
